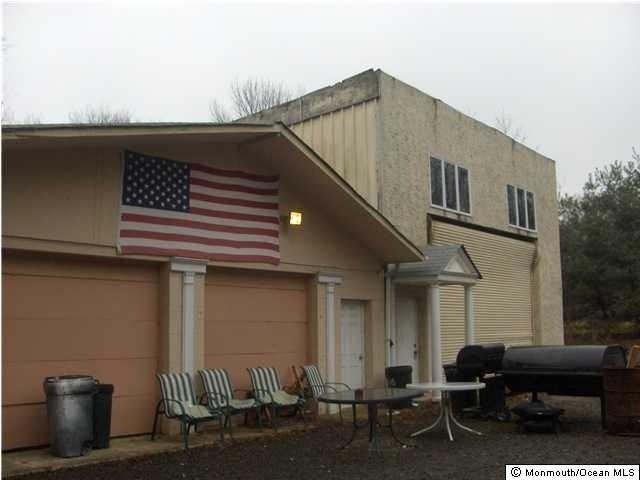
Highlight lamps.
[283,209,304,227]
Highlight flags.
[113,145,282,271]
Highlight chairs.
[150,364,353,448]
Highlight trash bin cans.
[43,374,114,457]
[385,366,412,409]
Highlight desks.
[318,380,488,451]
